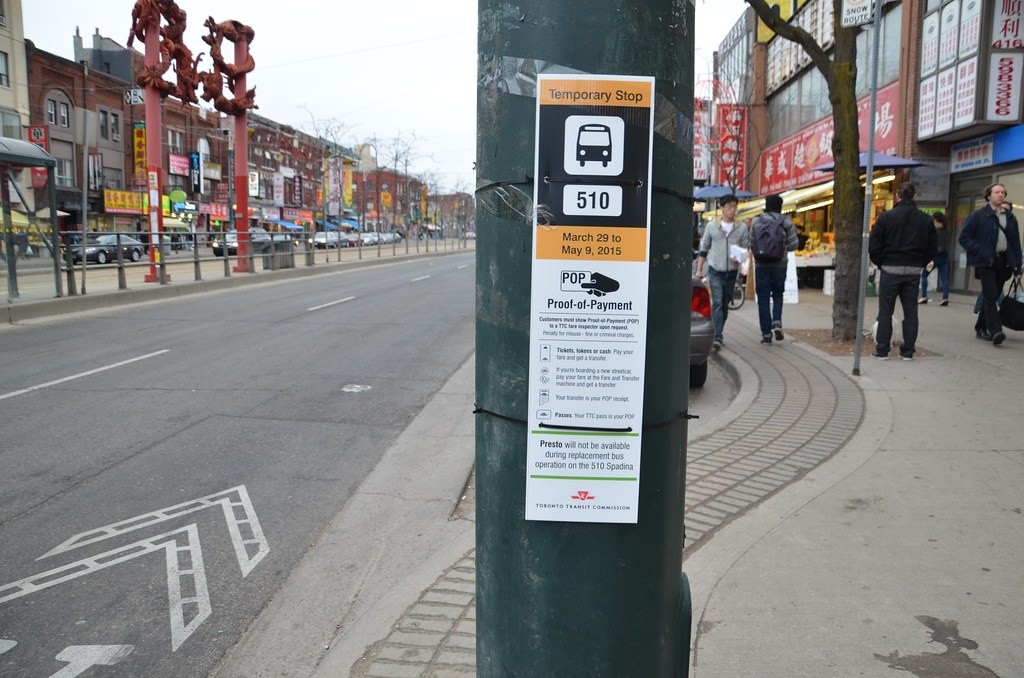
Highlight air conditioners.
[112,134,120,141]
[108,182,115,189]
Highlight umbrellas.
[26,207,70,222]
[693,184,758,201]
[810,150,928,173]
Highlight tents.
[0,207,53,234]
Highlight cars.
[690,275,714,387]
[308,230,402,249]
[63,234,146,264]
[273,235,300,251]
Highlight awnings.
[141,216,189,228]
[269,220,304,230]
[316,219,339,230]
[332,218,364,229]
[735,170,884,220]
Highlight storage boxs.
[865,281,875,297]
[824,269,834,296]
[795,256,832,267]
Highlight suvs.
[212,227,272,256]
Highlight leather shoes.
[976,328,991,340]
[992,326,1005,345]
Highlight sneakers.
[898,352,913,361]
[712,339,721,351]
[761,335,772,346]
[871,350,888,360]
[774,324,784,340]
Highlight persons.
[695,194,749,351]
[868,181,939,361]
[917,210,949,305]
[958,183,1024,347]
[748,194,799,346]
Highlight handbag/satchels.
[998,276,1024,331]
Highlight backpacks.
[754,214,786,264]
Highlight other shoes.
[918,297,927,305]
[939,299,949,306]
